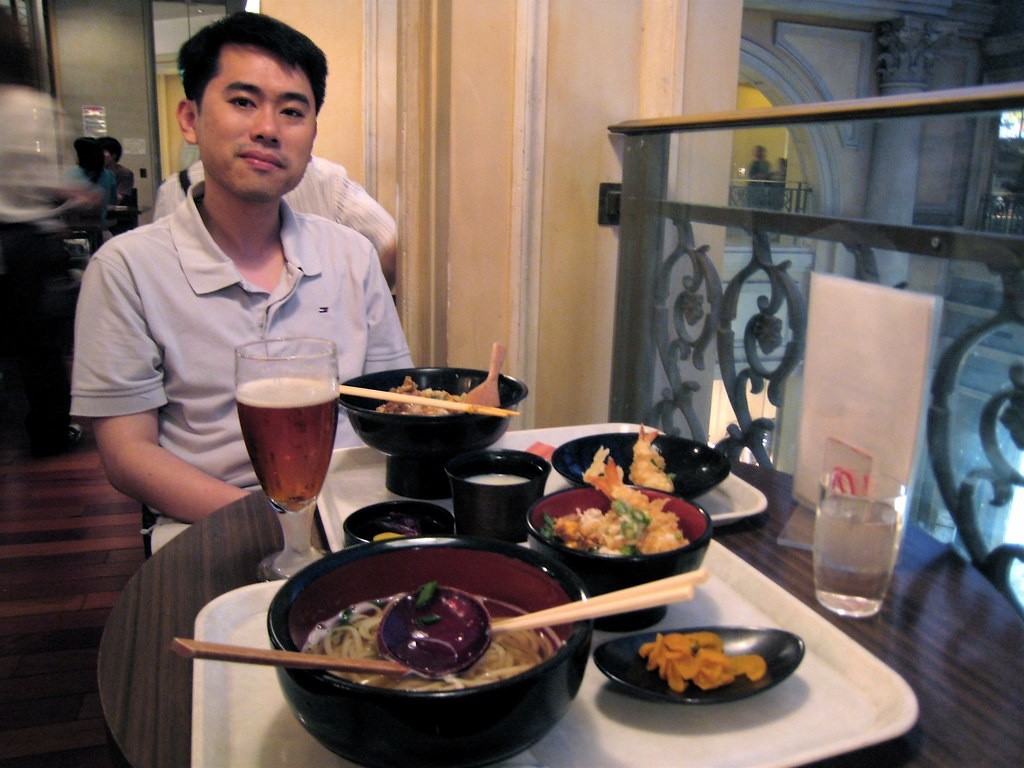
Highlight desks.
[97,461,1024,768]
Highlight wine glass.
[232,336,340,583]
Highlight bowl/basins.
[593,626,805,705]
[342,499,455,547]
[265,535,594,768]
[337,367,530,500]
[525,484,713,634]
[551,432,731,498]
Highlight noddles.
[302,591,564,688]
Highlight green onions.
[337,580,441,634]
[538,499,651,556]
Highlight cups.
[445,448,552,545]
[812,467,909,619]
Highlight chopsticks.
[339,383,522,420]
[490,568,711,635]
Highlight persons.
[66,136,118,220]
[0,8,98,460]
[151,154,398,260]
[70,12,415,562]
[96,137,135,203]
[744,145,786,238]
